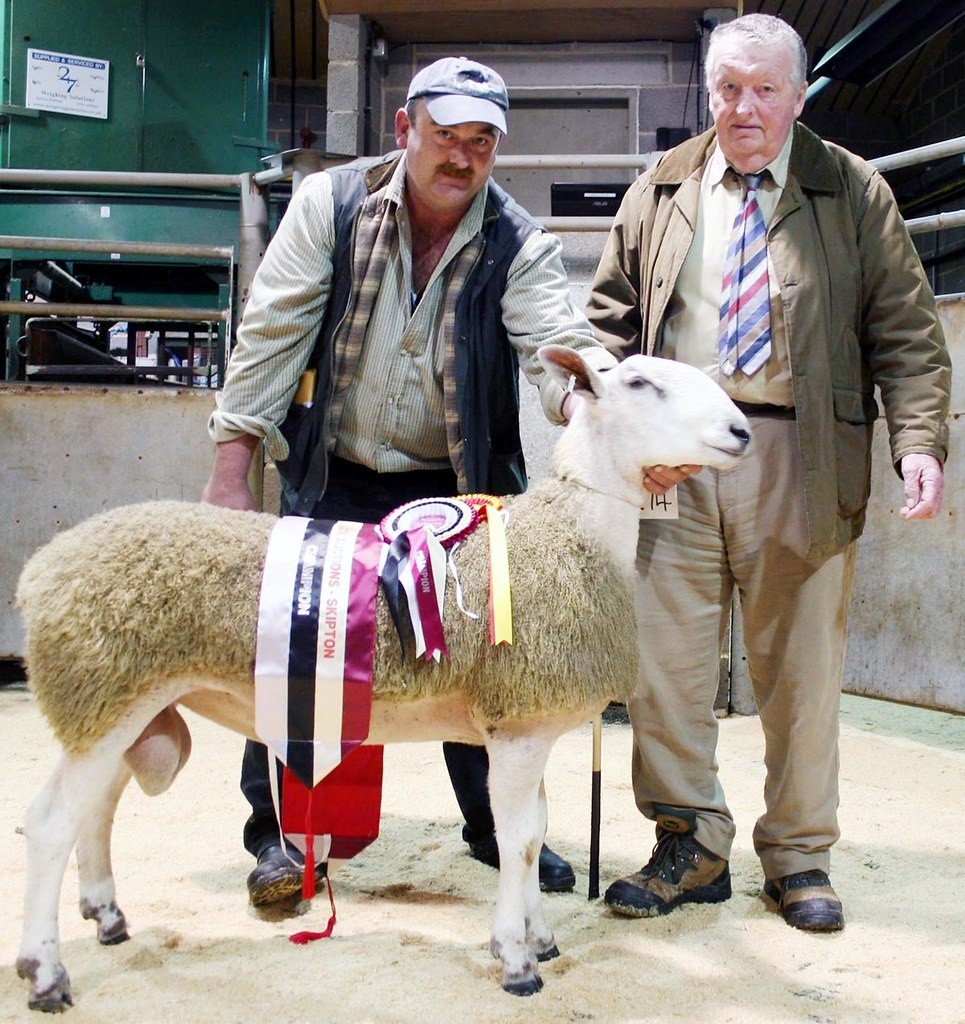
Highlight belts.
[730,401,787,415]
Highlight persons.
[583,13,954,931]
[198,53,701,909]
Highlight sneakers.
[762,866,842,930]
[606,802,730,917]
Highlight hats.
[407,55,509,137]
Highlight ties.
[717,169,773,376]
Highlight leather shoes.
[247,840,324,906]
[469,835,574,891]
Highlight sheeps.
[16,343,753,1014]
